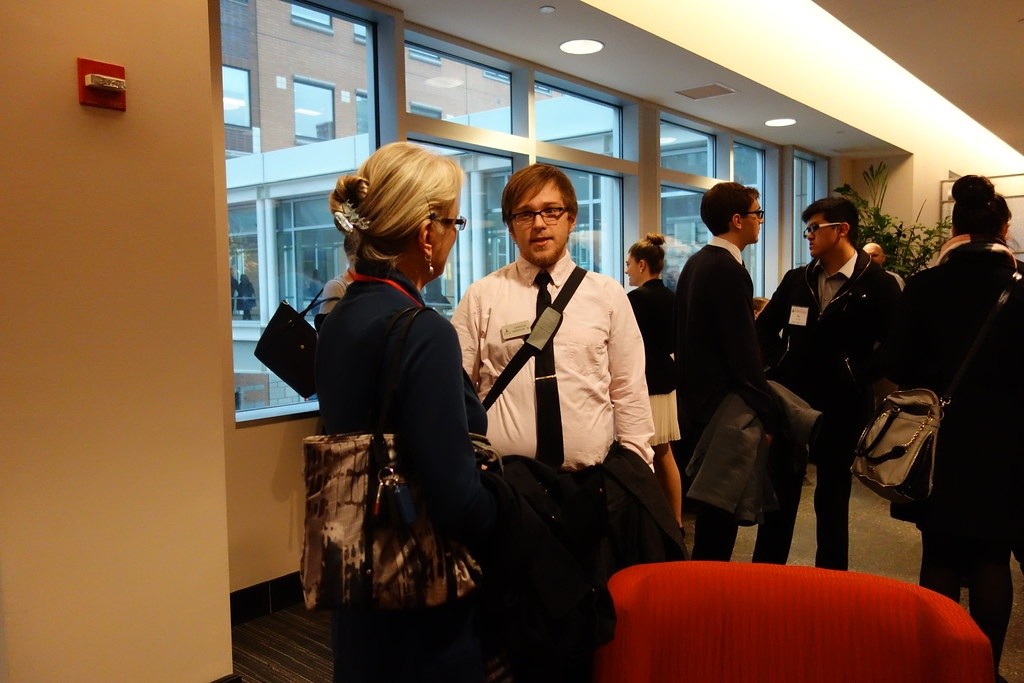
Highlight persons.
[863,242,904,293]
[731,197,909,571]
[873,176,1024,683]
[229,268,238,313]
[624,236,688,539]
[752,296,770,320]
[449,166,655,634]
[235,273,256,320]
[309,269,324,317]
[315,140,613,683]
[675,181,778,562]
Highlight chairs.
[592,560,994,683]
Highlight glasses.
[737,210,765,218]
[506,207,569,226]
[802,222,843,239]
[432,216,467,231]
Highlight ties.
[535,273,564,468]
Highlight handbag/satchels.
[299,306,505,613]
[849,385,942,502]
[253,286,341,398]
